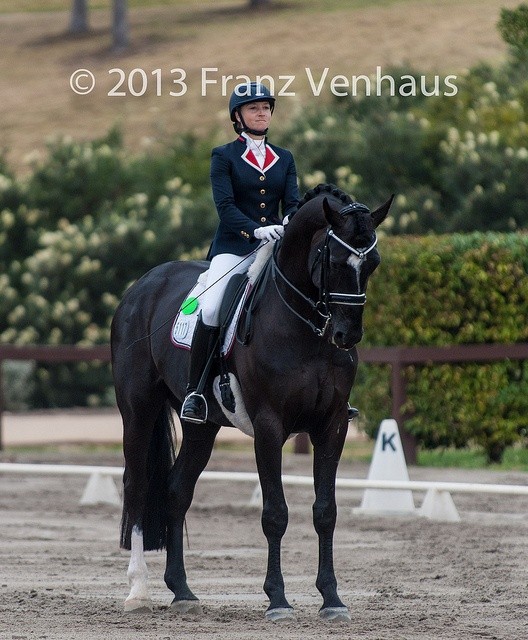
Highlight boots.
[348,403,359,419]
[183,309,221,418]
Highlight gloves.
[283,214,290,225]
[254,225,285,242]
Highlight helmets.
[229,82,274,134]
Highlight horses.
[109,182,397,621]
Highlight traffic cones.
[351,418,419,517]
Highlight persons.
[181,82,359,421]
